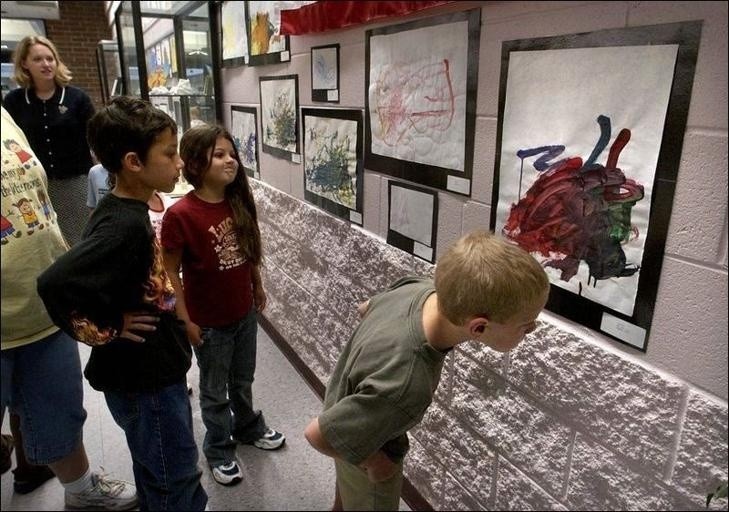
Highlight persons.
[35,97,210,511]
[162,122,285,485]
[0,106,138,511]
[305,230,551,512]
[1,404,57,494]
[6,34,94,252]
[85,151,116,216]
[138,189,193,394]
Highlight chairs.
[65,472,138,511]
[230,426,285,450]
[210,459,243,486]
[12,465,56,494]
[0,434,13,473]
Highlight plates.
[95,1,221,136]
